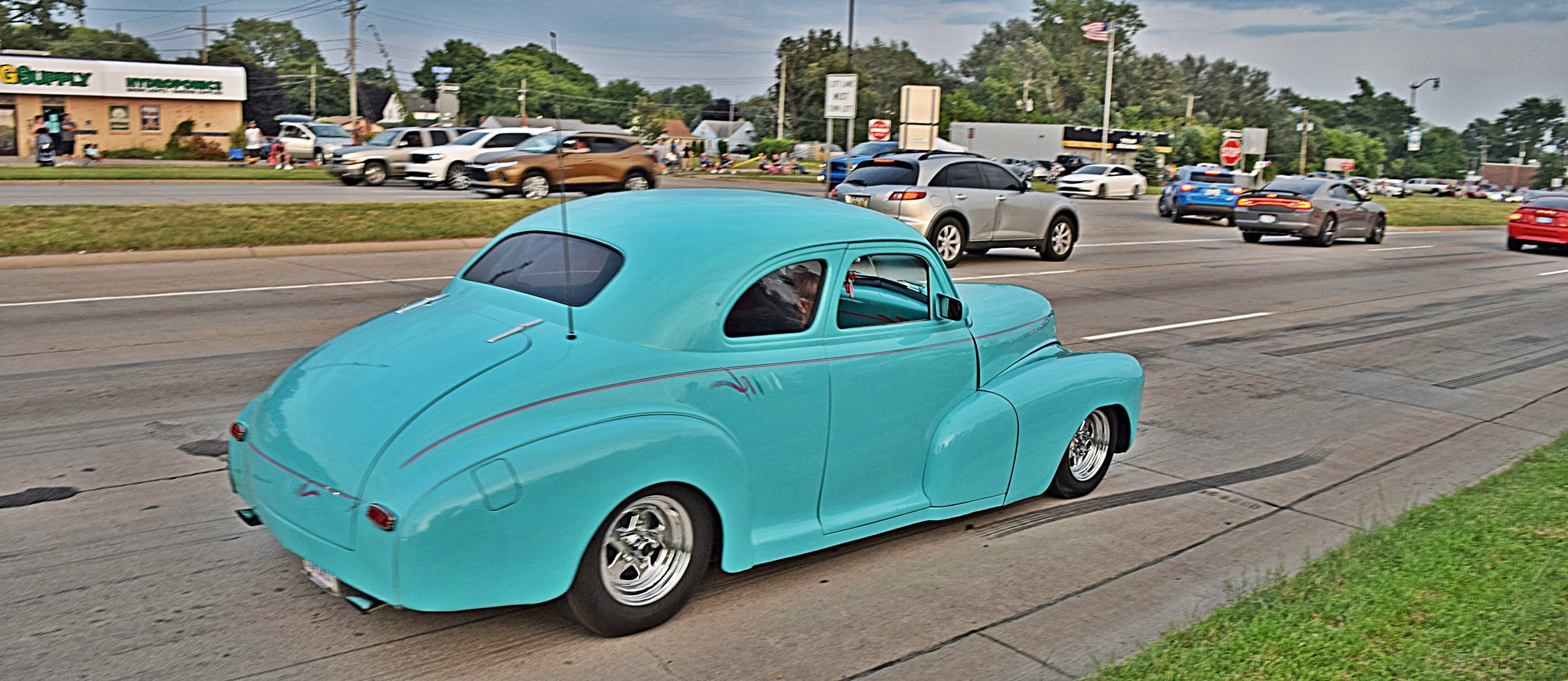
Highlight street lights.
[1399,77,1441,197]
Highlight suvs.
[830,149,1080,270]
[323,126,482,187]
[467,131,660,201]
[1157,165,1243,225]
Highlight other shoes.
[284,164,294,170]
[275,163,282,169]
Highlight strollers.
[36,133,57,167]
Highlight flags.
[1079,20,1111,43]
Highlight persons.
[759,152,810,175]
[699,151,721,174]
[562,139,590,153]
[651,137,694,174]
[474,115,487,129]
[29,115,50,138]
[351,121,363,146]
[84,143,109,164]
[60,113,79,157]
[244,120,263,164]
[719,153,737,174]
[273,141,294,170]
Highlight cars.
[271,114,356,166]
[1308,171,1529,203]
[226,188,1145,638]
[1057,163,1149,199]
[1506,197,1568,251]
[1233,176,1389,248]
[1000,155,1096,184]
[817,139,900,193]
[402,124,563,191]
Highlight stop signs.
[1220,139,1243,166]
[867,119,891,141]
